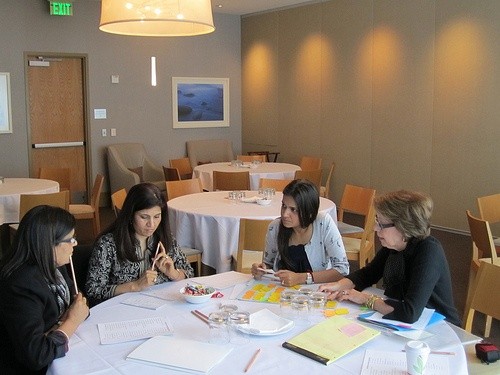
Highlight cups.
[207,312,231,345]
[280,288,327,323]
[405,341,428,375]
[228,190,245,201]
[258,188,275,199]
[218,304,238,329]
[229,311,250,344]
[230,159,244,168]
[250,159,262,169]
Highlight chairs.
[0,138,500,375]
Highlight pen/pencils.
[191,309,209,325]
[152,243,160,270]
[69,255,78,294]
[402,349,456,355]
[263,269,275,274]
[244,349,261,373]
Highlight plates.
[236,318,295,335]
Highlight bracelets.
[365,294,381,310]
[171,268,181,280]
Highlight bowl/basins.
[180,285,216,304]
[256,198,272,206]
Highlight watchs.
[306,272,314,284]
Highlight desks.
[44,272,469,375]
[166,190,338,282]
[189,160,302,193]
[0,178,60,232]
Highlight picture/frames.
[172,77,230,128]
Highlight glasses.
[375,214,395,231]
[55,233,77,244]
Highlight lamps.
[98,0,216,37]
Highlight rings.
[154,278,156,282]
[166,256,168,259]
[342,291,345,295]
[281,280,284,285]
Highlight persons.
[317,189,462,329]
[85,183,194,308]
[251,180,350,287]
[0,205,89,375]
